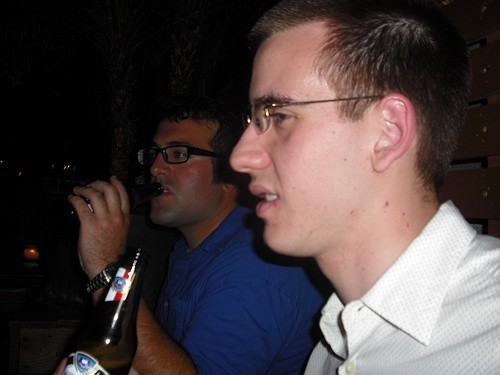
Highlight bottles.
[62,246,149,375]
[59,182,164,229]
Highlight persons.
[68,98,327,375]
[52,0,500,375]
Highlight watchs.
[88,262,117,295]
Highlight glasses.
[242,94,386,136]
[137,144,230,169]
